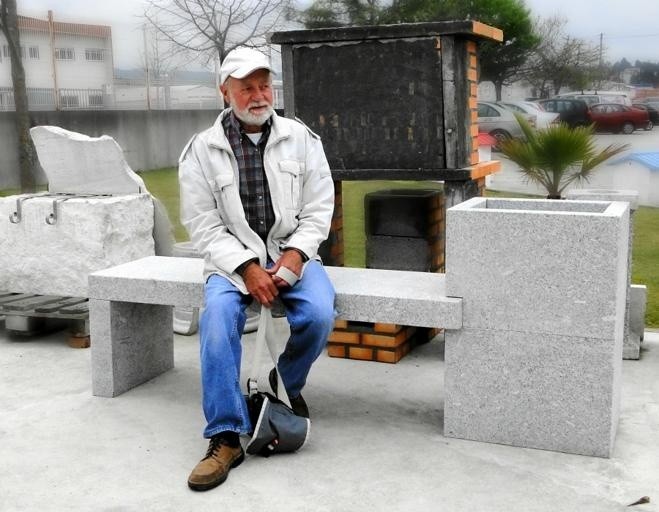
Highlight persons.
[176,46,342,490]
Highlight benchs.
[86,253,467,405]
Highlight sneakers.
[267,367,309,417]
[187,435,245,492]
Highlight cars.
[475,87,659,151]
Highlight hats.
[218,47,277,85]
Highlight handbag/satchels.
[244,391,312,457]
